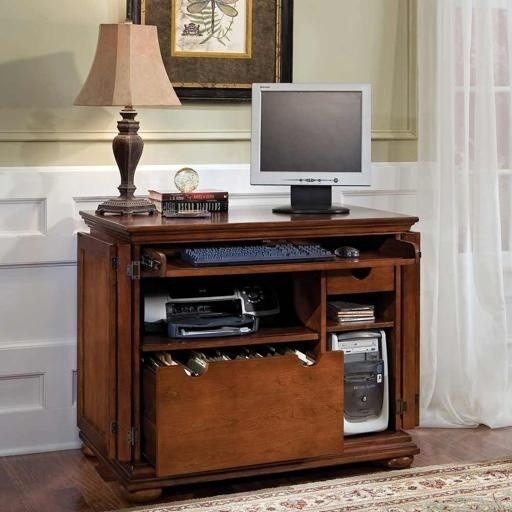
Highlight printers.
[142,278,283,339]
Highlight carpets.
[116,456,512,512]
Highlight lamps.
[72,19,181,214]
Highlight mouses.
[336,247,361,259]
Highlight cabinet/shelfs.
[75,205,421,502]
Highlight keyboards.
[180,242,334,266]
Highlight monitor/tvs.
[249,82,372,215]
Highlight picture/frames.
[126,0,293,103]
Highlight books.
[327,301,375,326]
[148,189,228,201]
[149,201,228,214]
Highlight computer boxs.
[328,331,390,439]
